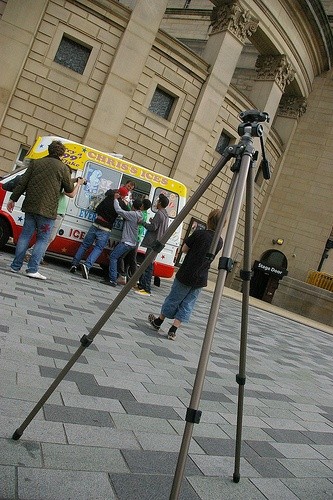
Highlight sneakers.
[24,254,32,262]
[148,314,161,331]
[135,289,151,296]
[26,271,47,280]
[168,332,176,341]
[100,280,117,287]
[132,287,140,291]
[70,266,77,273]
[11,268,17,273]
[116,275,126,285]
[81,264,89,279]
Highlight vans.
[0,135,188,286]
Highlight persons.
[149,209,227,340]
[131,194,169,296]
[23,164,85,264]
[71,180,151,289]
[7,141,74,280]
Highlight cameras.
[76,177,87,185]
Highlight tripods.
[12,110,271,500]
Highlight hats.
[118,187,128,197]
